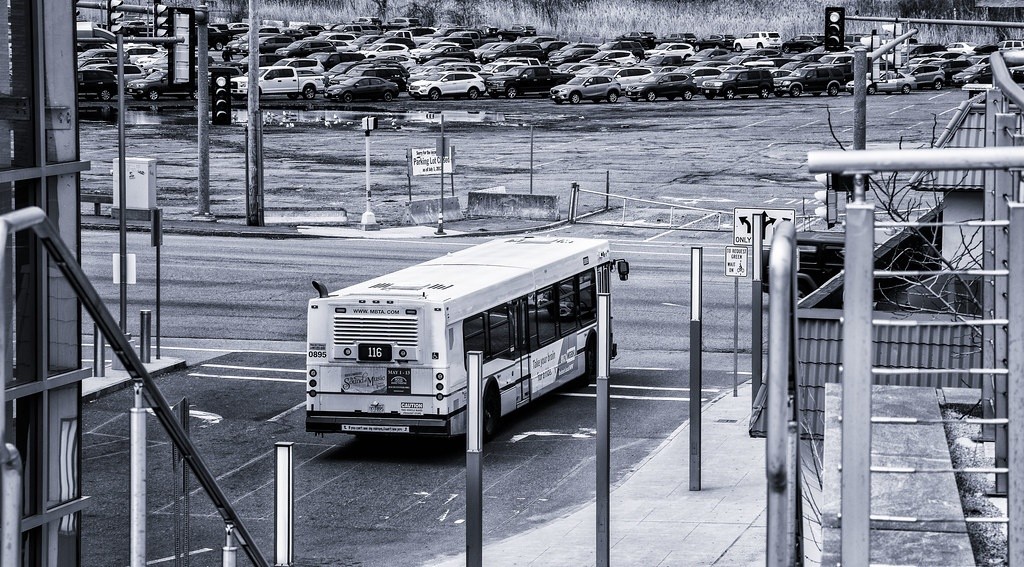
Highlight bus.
[304,236,631,456]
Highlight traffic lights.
[814,173,826,222]
[110,0,125,35]
[860,36,872,49]
[213,73,230,125]
[883,22,893,67]
[368,117,378,132]
[827,7,843,52]
[155,3,169,36]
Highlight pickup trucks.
[230,66,326,101]
[485,66,576,99]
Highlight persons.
[223,47,235,60]
[578,36,582,43]
[375,20,382,35]
[602,38,605,45]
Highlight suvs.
[613,31,736,52]
[732,32,782,52]
[78,44,222,101]
[773,64,846,97]
[700,68,774,100]
[783,31,872,54]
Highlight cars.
[624,73,698,102]
[470,36,1024,89]
[847,71,918,95]
[194,17,536,92]
[407,71,486,101]
[549,75,621,105]
[323,76,400,103]
